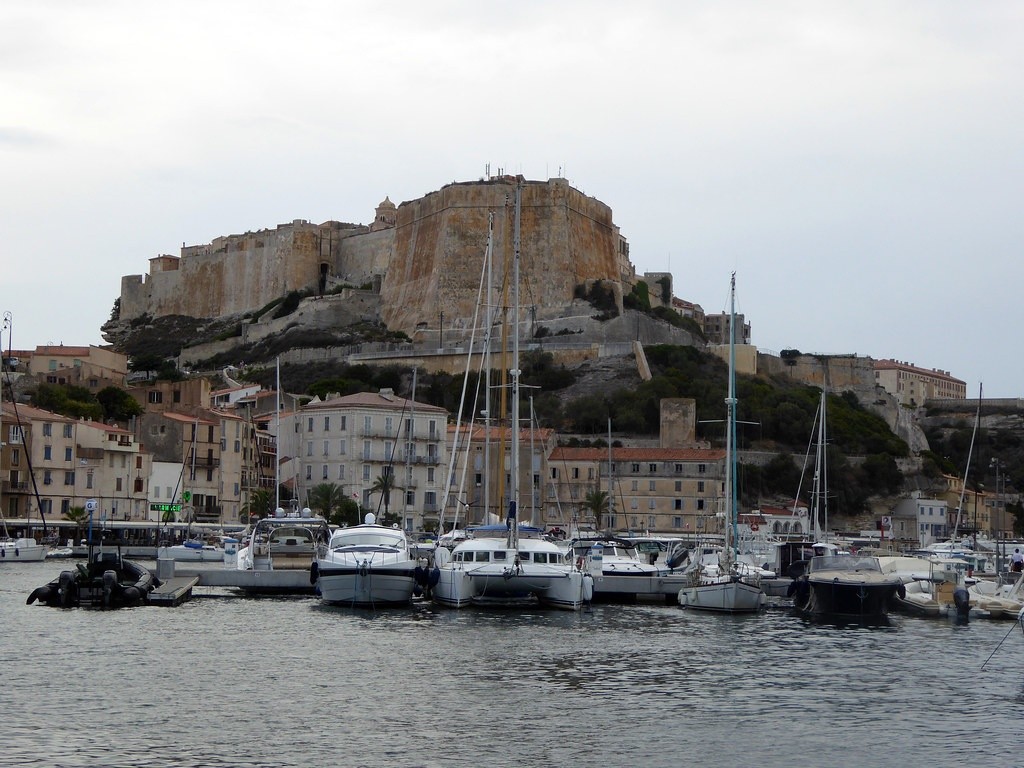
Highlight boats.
[236,507,333,593]
[967,571,1024,620]
[894,557,1004,620]
[0,538,50,562]
[25,499,160,611]
[788,554,906,625]
[915,535,1024,574]
[310,513,424,607]
[157,539,225,562]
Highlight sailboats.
[677,269,768,614]
[408,213,851,597]
[423,175,596,611]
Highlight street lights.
[989,457,1007,576]
[997,473,1011,573]
[3,311,12,372]
